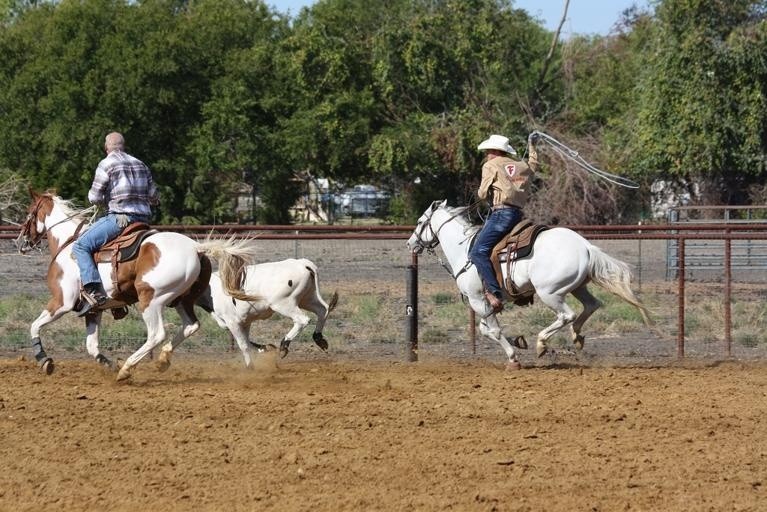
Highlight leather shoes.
[481,298,507,319]
[77,291,106,317]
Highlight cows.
[191,256,341,372]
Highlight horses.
[13,182,268,383]
[404,197,654,373]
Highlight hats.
[478,134,517,155]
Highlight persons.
[70,131,160,318]
[468,130,544,319]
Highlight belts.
[493,204,518,209]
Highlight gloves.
[529,130,539,142]
